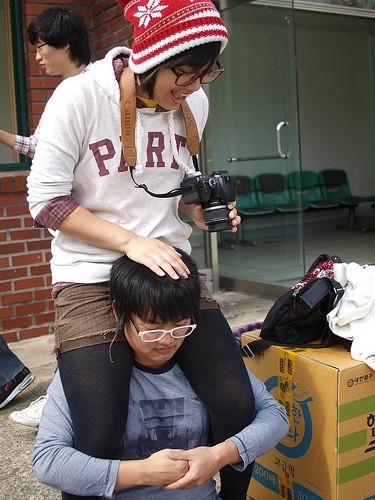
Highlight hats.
[118,0,228,74]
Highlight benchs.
[179,169,375,252]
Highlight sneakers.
[0,366,35,409]
[9,396,46,426]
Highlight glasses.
[167,61,225,85]
[33,43,48,56]
[127,315,197,343]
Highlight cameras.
[180,170,236,232]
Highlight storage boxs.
[241,326,375,500]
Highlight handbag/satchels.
[259,254,343,344]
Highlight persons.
[0,337,35,409]
[26,0,256,500]
[31,248,290,500]
[0,7,94,428]
